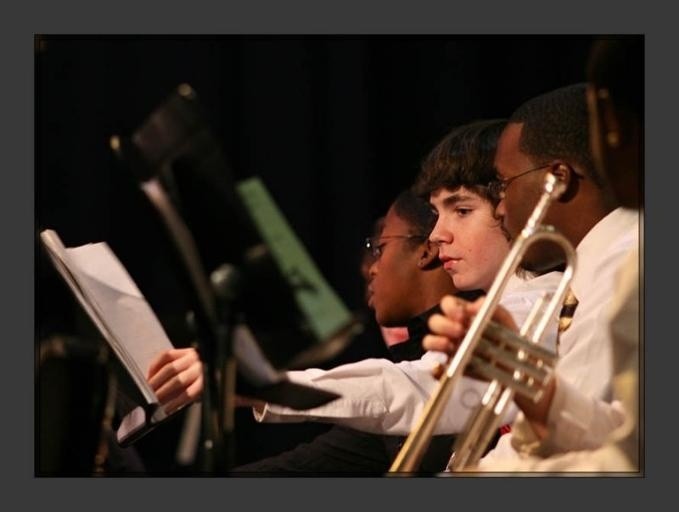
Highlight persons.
[145,84,645,479]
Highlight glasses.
[487,163,585,199]
[362,235,429,257]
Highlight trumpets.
[389,173,578,475]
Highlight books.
[41,228,177,405]
